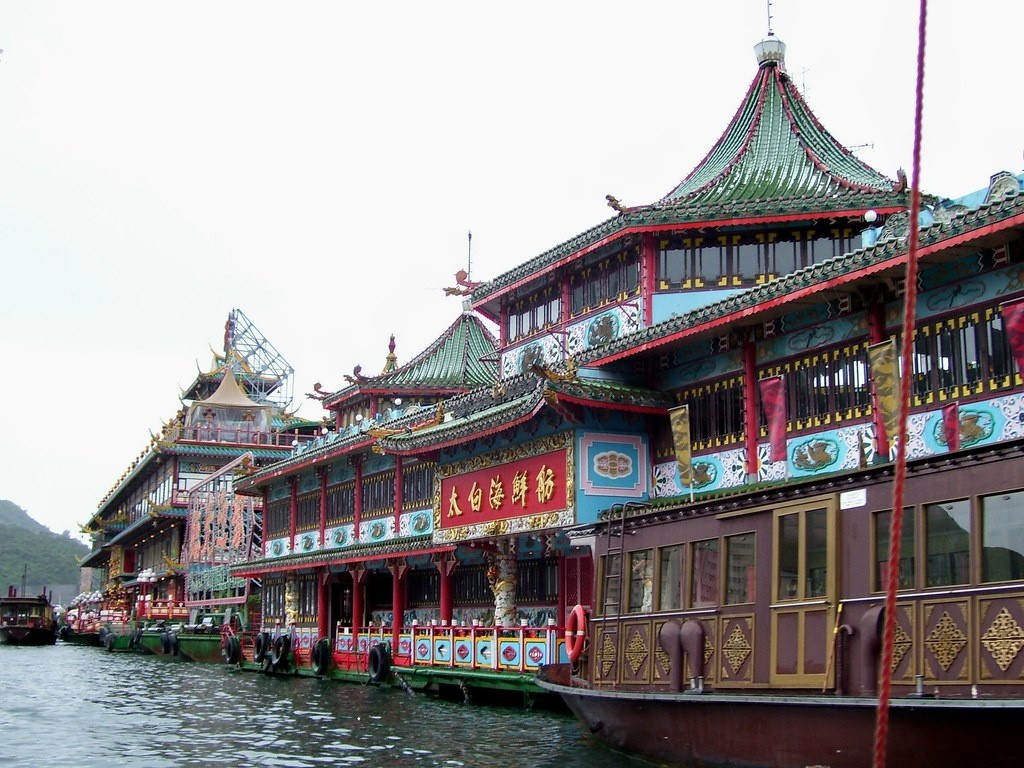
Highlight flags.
[942,403,958,452]
[1002,303,1024,384]
[759,377,786,462]
[669,408,691,485]
[869,342,901,441]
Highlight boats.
[0,564,59,647]
[530,0,1024,768]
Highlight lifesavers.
[105,633,114,652]
[127,633,134,648]
[366,644,390,682]
[168,634,178,657]
[224,637,241,664]
[271,635,290,667]
[562,605,587,661]
[159,633,169,655]
[133,630,143,645]
[252,632,268,663]
[310,639,331,677]
